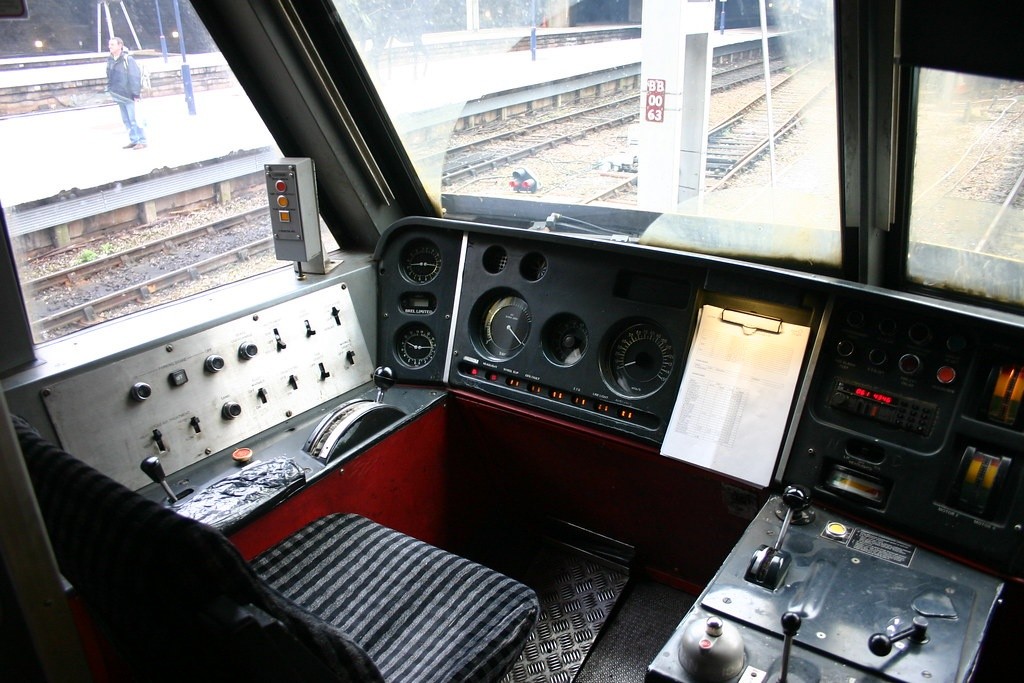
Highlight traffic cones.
[542,16,548,28]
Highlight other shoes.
[123,142,147,150]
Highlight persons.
[106,37,150,151]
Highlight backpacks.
[123,54,151,91]
